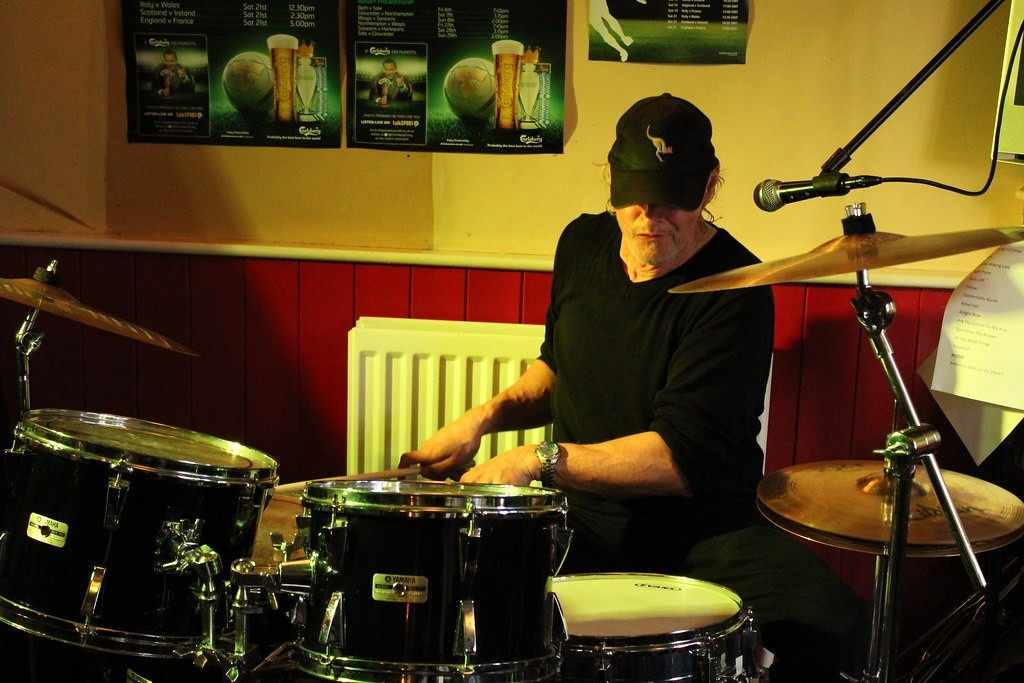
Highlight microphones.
[754,175,882,213]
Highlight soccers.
[223,51,276,113]
[442,57,496,120]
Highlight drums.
[550,569,764,683]
[228,493,315,637]
[1,408,281,660]
[294,470,567,683]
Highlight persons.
[371,59,409,106]
[153,50,191,99]
[397,93,840,683]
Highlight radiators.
[345,316,774,488]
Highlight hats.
[607,93,719,210]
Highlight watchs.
[534,440,561,487]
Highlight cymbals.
[756,459,1024,558]
[0,278,203,358]
[668,227,1024,295]
